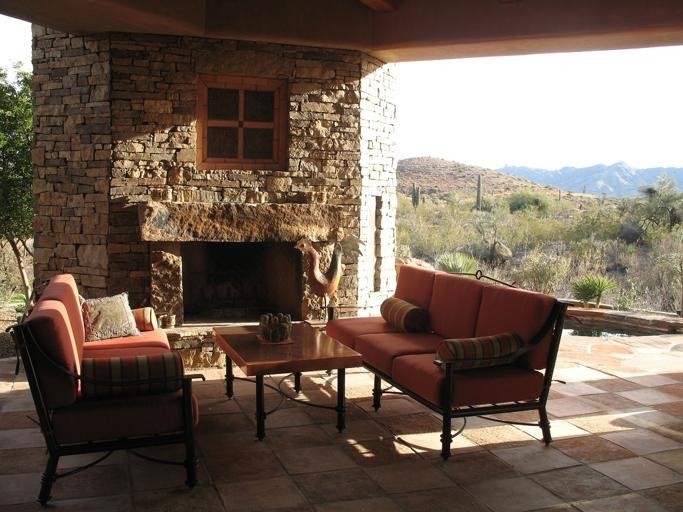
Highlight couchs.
[320,264,576,461]
[4,272,207,507]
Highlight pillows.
[79,290,142,342]
